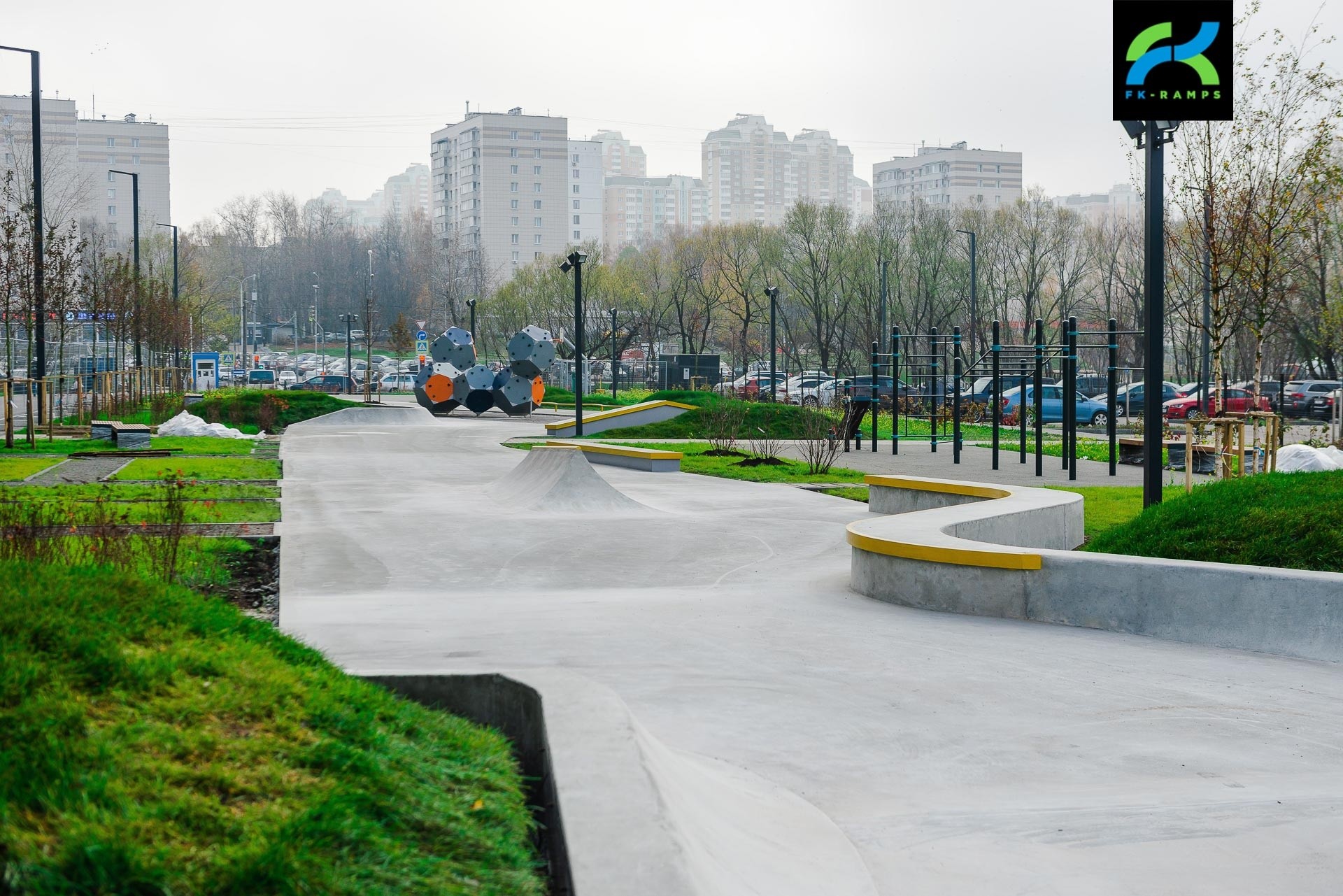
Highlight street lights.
[882,260,892,375]
[0,45,45,425]
[156,223,181,393]
[763,287,780,403]
[608,308,617,400]
[109,169,143,408]
[225,275,256,370]
[313,285,320,377]
[1120,119,1182,508]
[466,299,477,347]
[367,249,373,382]
[431,276,468,331]
[559,251,588,437]
[339,312,359,395]
[955,229,975,388]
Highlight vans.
[1055,375,1108,398]
[837,375,917,410]
[945,376,1055,405]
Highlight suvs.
[1276,380,1343,421]
[285,330,374,344]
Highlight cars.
[487,361,505,371]
[759,371,848,408]
[986,384,1118,426]
[424,332,436,337]
[1090,381,1183,417]
[712,360,792,400]
[589,361,659,381]
[218,352,432,393]
[1162,380,1288,420]
[0,368,29,380]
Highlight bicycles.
[932,394,982,423]
